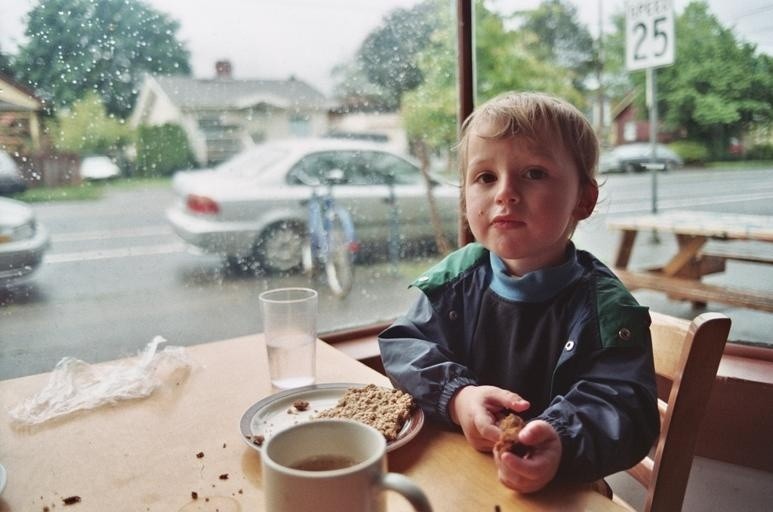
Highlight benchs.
[616,254,772,310]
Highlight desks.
[604,211,773,472]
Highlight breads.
[318,383,416,442]
[494,413,527,458]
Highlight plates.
[239,383,426,455]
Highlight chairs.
[594,309,731,512]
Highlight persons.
[377,90,661,501]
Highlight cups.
[258,287,318,389]
[259,420,434,512]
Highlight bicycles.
[302,169,357,299]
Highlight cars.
[79,154,121,184]
[172,137,462,277]
[600,143,681,176]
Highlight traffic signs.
[624,3,676,73]
